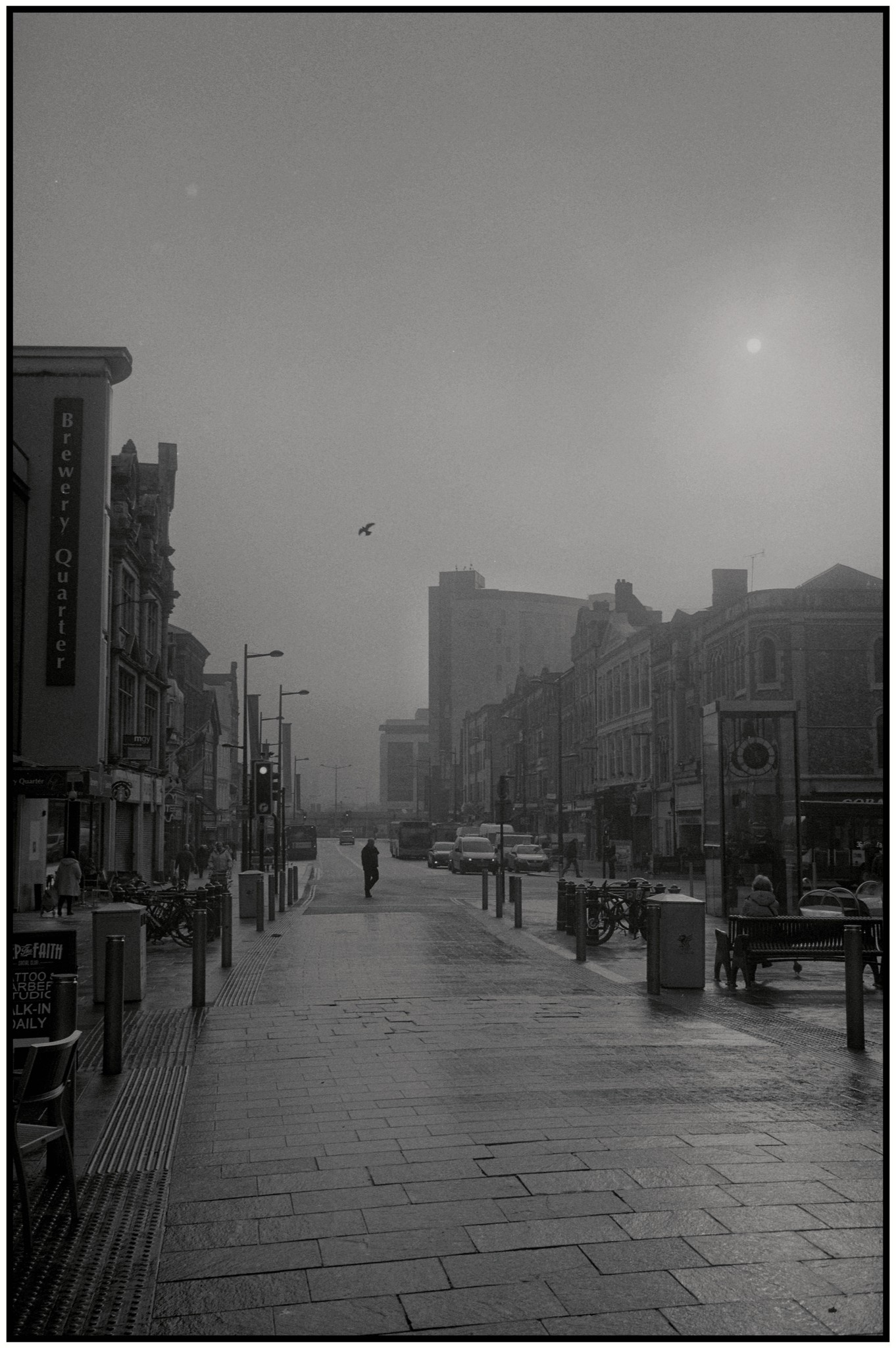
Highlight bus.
[431,820,463,842]
[388,820,433,859]
[284,824,318,860]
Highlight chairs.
[12,1028,83,1240]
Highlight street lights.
[293,755,309,824]
[258,685,309,875]
[321,764,353,828]
[356,787,368,838]
[530,678,566,879]
[222,643,284,873]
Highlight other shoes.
[747,971,755,986]
[763,962,774,968]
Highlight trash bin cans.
[91,903,147,1003]
[239,870,265,918]
[240,853,255,870]
[646,894,707,992]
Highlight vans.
[449,822,525,874]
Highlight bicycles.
[583,877,654,945]
[114,877,221,949]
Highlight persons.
[868,850,883,895]
[863,822,883,845]
[607,840,616,880]
[560,838,585,878]
[172,839,236,889]
[54,849,82,916]
[493,842,505,876]
[246,846,275,872]
[361,837,380,899]
[740,875,780,991]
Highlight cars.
[507,844,550,873]
[427,842,456,869]
[338,830,355,845]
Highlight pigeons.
[792,960,803,977]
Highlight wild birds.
[358,522,375,537]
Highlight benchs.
[714,915,883,991]
